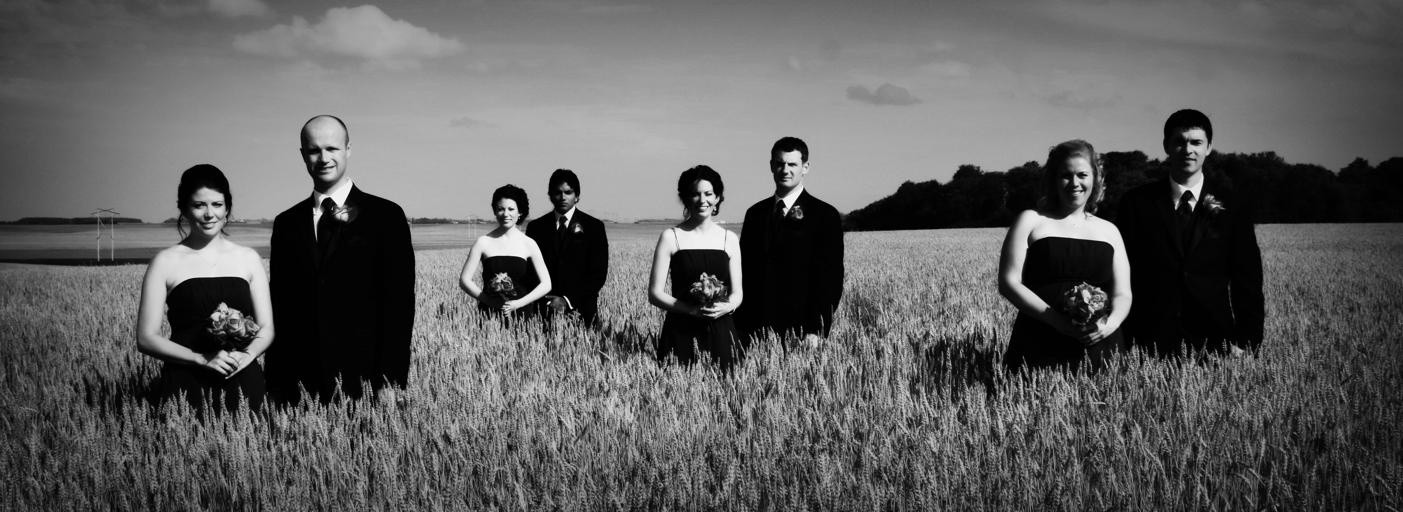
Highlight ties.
[775,199,786,221]
[317,197,339,259]
[557,216,567,232]
[1177,191,1191,222]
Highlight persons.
[647,162,745,379]
[524,168,608,334]
[996,139,1133,379]
[1120,107,1265,369]
[458,185,551,329]
[134,162,277,422]
[736,137,844,354]
[262,114,415,413]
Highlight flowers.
[1063,281,1108,330]
[491,272,517,302]
[208,301,261,352]
[690,272,728,308]
